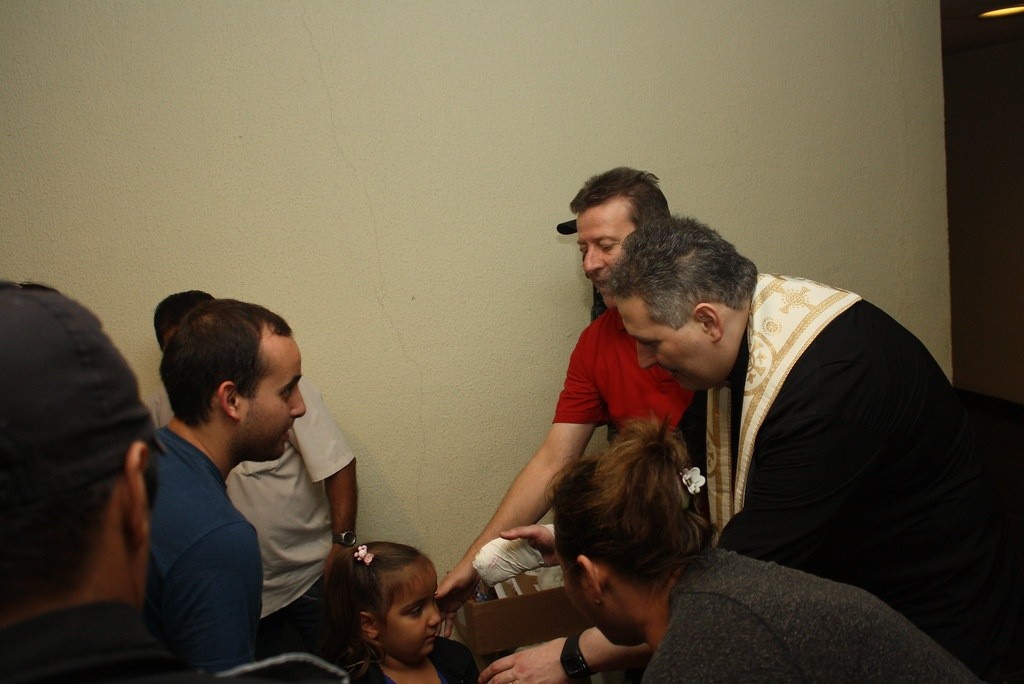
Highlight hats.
[0,290,167,548]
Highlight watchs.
[560,634,596,678]
[333,530,356,546]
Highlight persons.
[0,282,483,684]
[429,166,1024,684]
